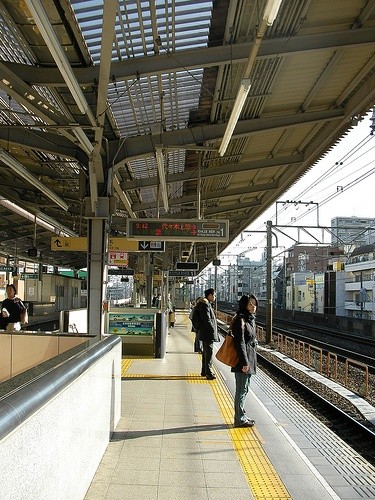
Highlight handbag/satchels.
[215,335,241,368]
[20,312,28,324]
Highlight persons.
[193,288,220,379]
[189,297,204,354]
[230,292,258,428]
[1,284,28,330]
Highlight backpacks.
[189,306,199,332]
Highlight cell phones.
[248,369,251,374]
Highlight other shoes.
[201,373,216,380]
[193,350,202,354]
[233,420,255,427]
